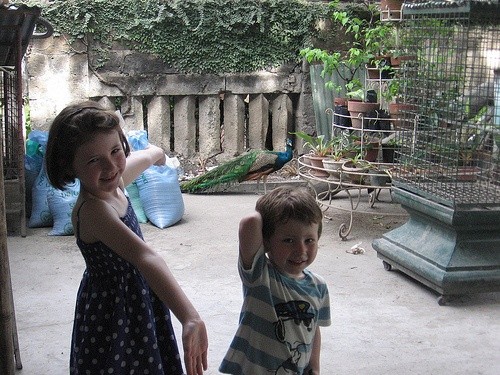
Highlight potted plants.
[334,0,416,79]
[288,129,486,185]
[324,79,420,129]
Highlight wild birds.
[180,137,294,197]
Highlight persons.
[45,102,208,375]
[218,185,332,375]
